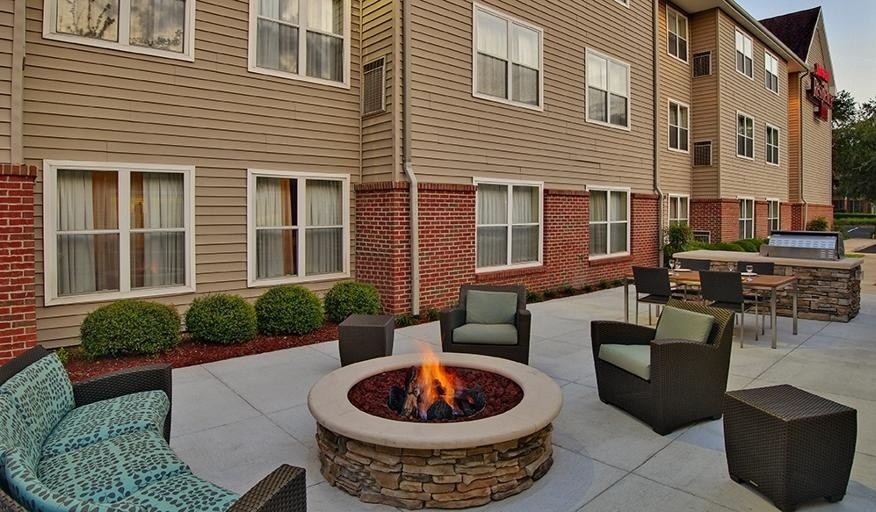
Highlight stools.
[725,384,857,512]
[338,314,395,367]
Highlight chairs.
[735,261,775,335]
[632,265,685,328]
[698,270,758,347]
[675,259,711,303]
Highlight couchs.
[0,345,306,510]
[590,299,736,436]
[439,284,531,365]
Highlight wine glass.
[668,259,676,274]
[727,262,735,272]
[675,261,681,275]
[746,265,754,282]
